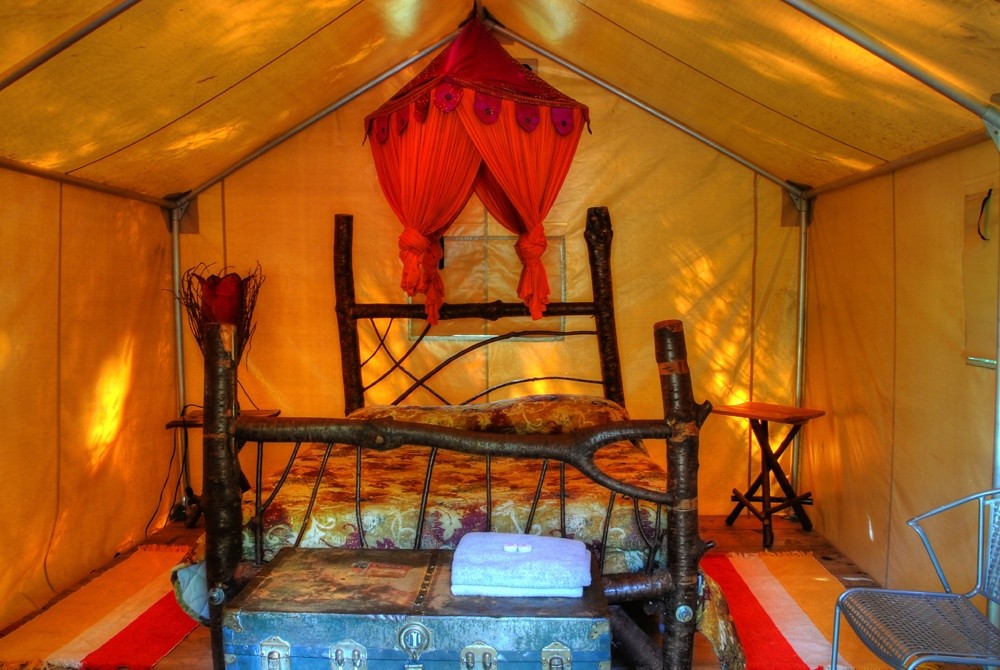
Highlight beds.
[209,206,715,670]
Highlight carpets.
[697,552,938,670]
[0,545,205,670]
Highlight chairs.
[830,488,1000,670]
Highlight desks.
[164,406,283,530]
[709,390,827,546]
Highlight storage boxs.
[220,546,611,670]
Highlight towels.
[448,533,594,600]
